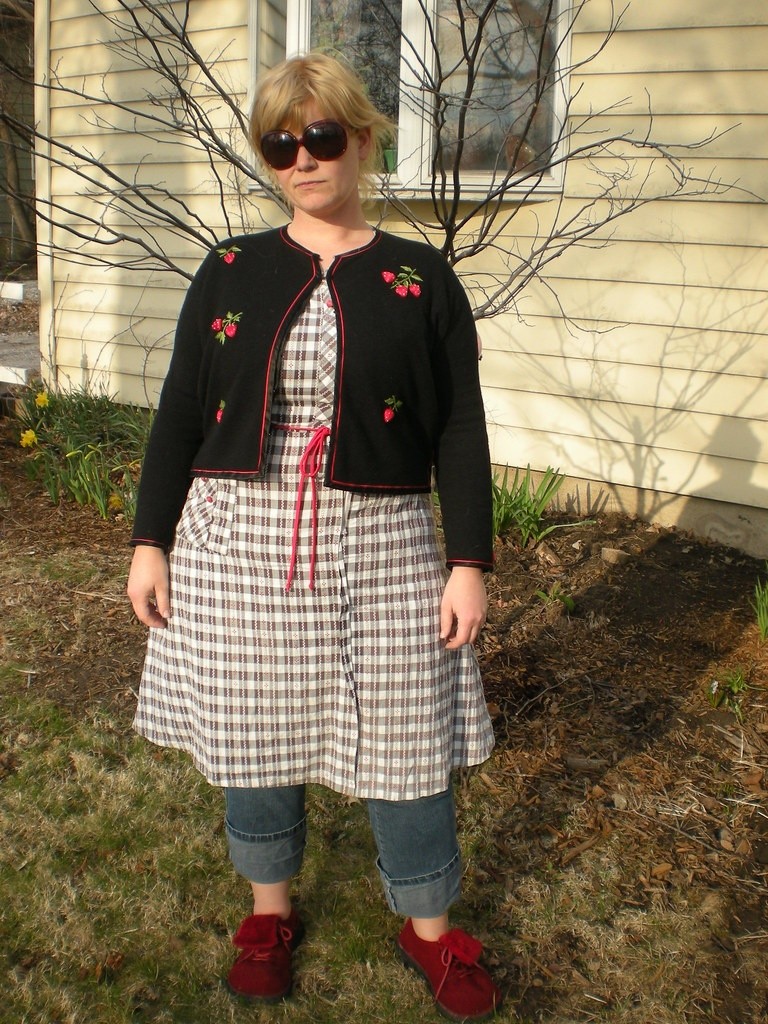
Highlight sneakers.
[395,913,502,1024]
[226,902,304,1004]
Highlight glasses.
[260,119,348,170]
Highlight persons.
[126,52,509,1024]
